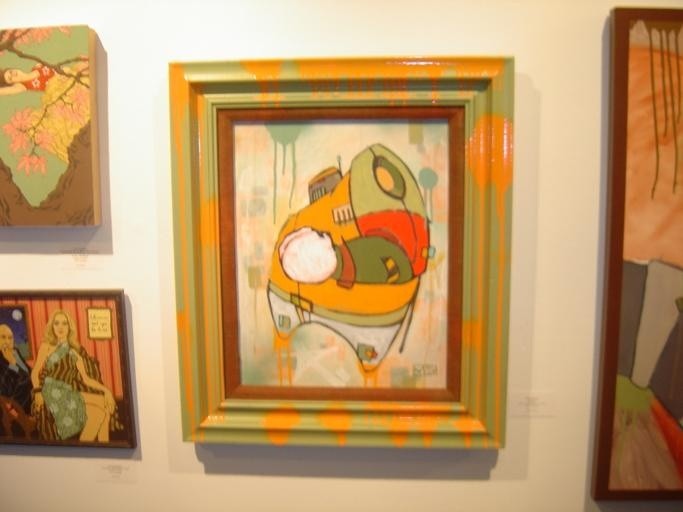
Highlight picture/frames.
[587,7,683,503]
[167,52,513,449]
[0,287,137,448]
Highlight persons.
[1,61,57,96]
[31,310,116,442]
[0,324,31,415]
